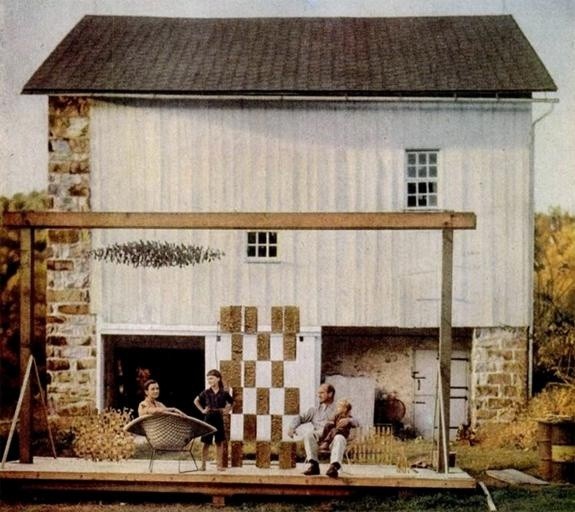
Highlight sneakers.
[326,466,338,478]
[304,464,320,475]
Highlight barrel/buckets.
[536,419,574,482]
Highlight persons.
[316,398,353,451]
[194,368,238,472]
[285,382,361,478]
[137,377,188,420]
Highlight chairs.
[123,410,218,473]
[301,431,357,474]
